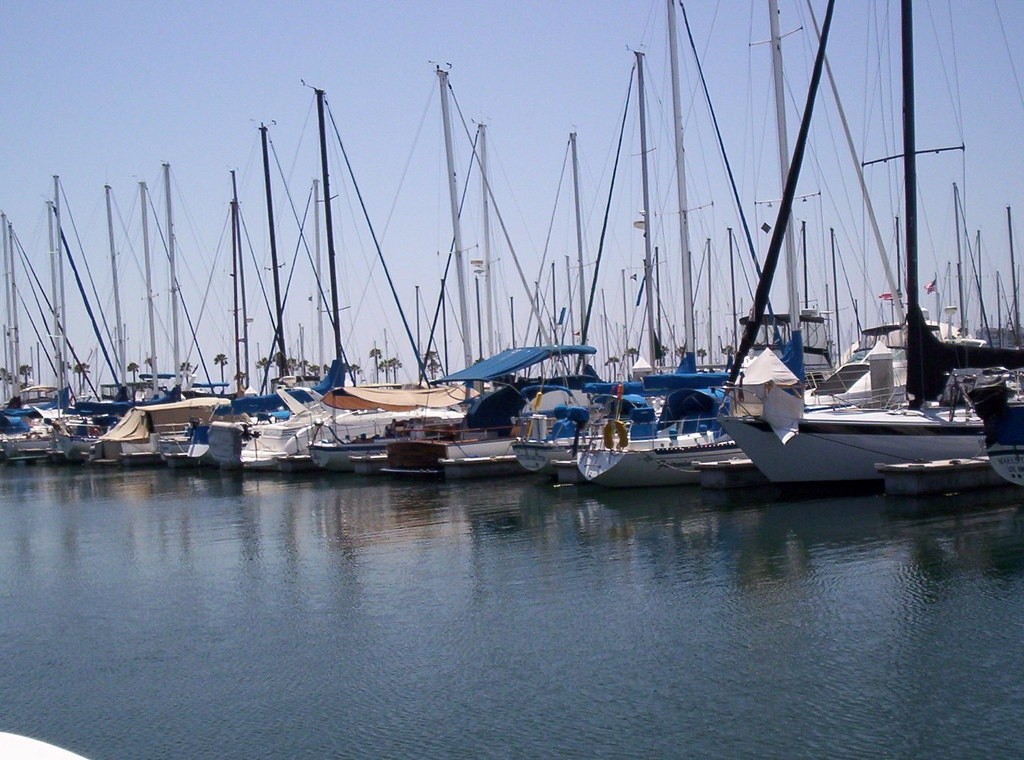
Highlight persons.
[510,420,533,438]
[343,418,425,444]
[89,426,101,437]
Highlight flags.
[878,289,903,300]
[924,279,937,295]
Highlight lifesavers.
[92,427,99,435]
[603,421,629,449]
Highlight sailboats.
[0,0,1024,503]
